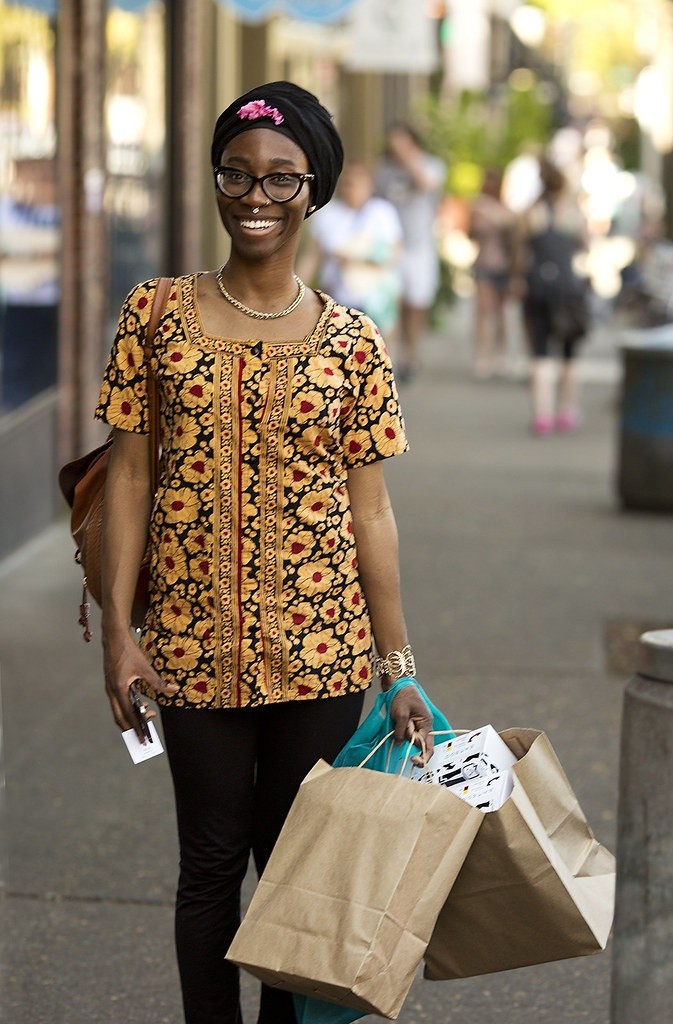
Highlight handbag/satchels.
[58,277,174,642]
[224,675,616,1024]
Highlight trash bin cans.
[614,342,673,513]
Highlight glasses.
[214,165,315,204]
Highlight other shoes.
[533,410,581,435]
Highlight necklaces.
[216,263,306,319]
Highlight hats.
[211,81,344,220]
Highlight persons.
[310,121,591,437]
[99,79,434,1024]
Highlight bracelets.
[372,645,416,679]
